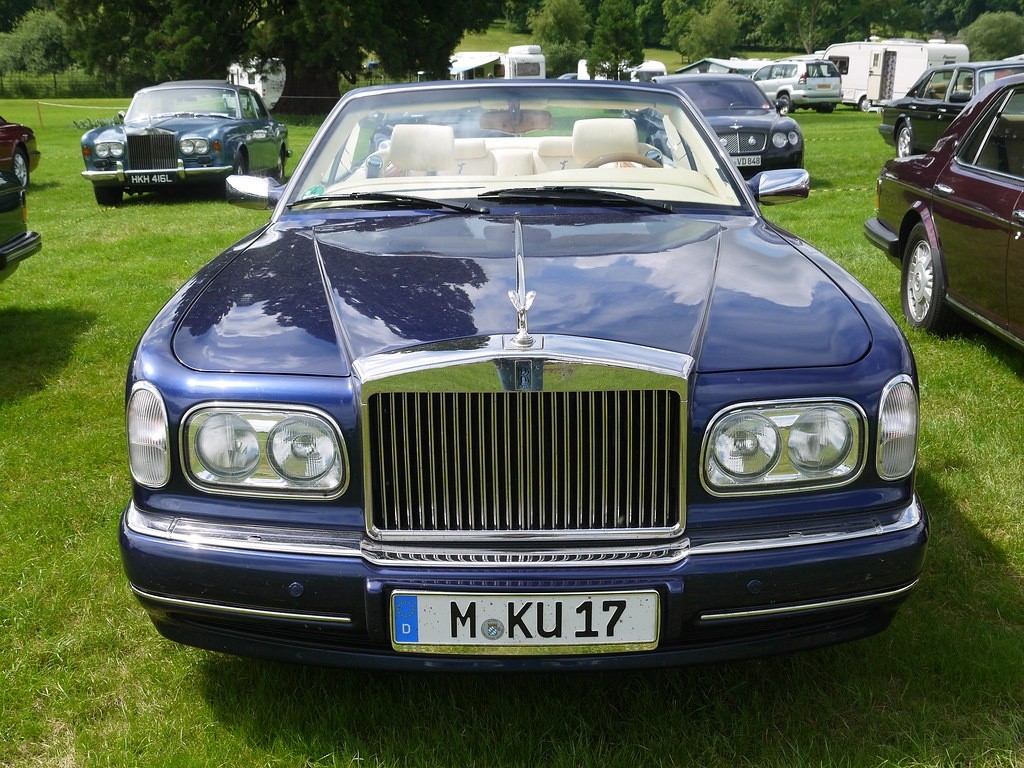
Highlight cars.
[78,79,293,207]
[878,60,1024,160]
[1,112,42,282]
[861,72,1024,354]
[115,76,935,675]
[647,75,805,180]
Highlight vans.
[821,34,844,113]
[816,35,969,114]
[417,44,667,82]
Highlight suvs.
[748,57,843,114]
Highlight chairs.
[962,76,985,93]
[572,117,639,168]
[389,120,455,176]
[975,113,1024,177]
[930,81,949,99]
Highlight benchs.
[439,137,574,177]
